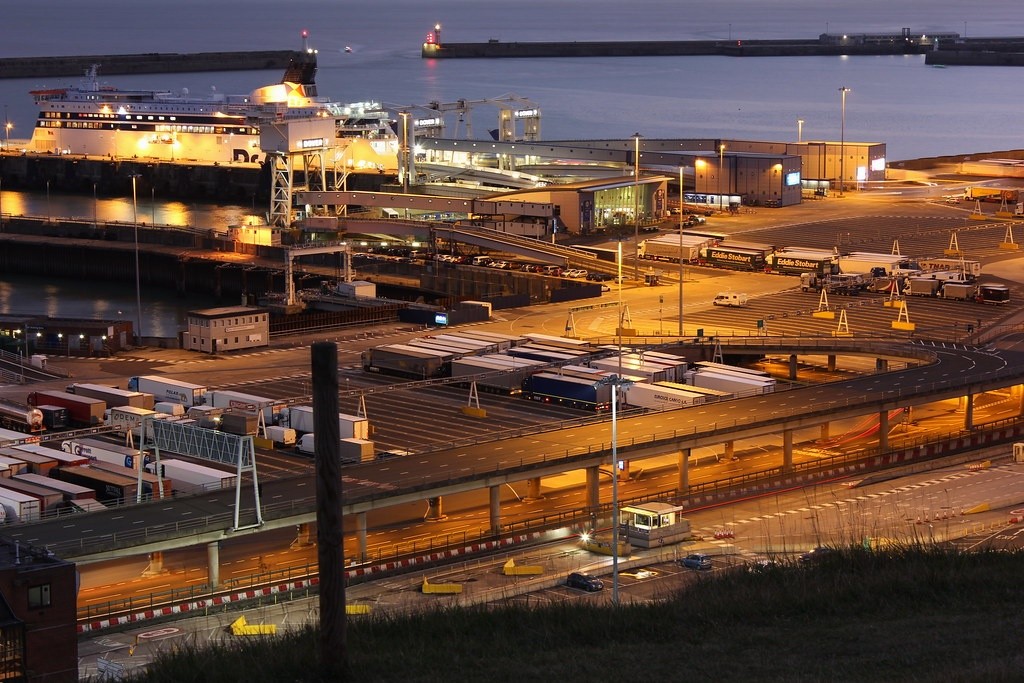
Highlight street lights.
[720,144,725,212]
[593,372,636,606]
[839,86,851,195]
[797,119,805,142]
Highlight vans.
[712,292,747,308]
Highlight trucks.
[799,265,979,302]
[0,372,375,526]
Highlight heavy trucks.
[962,187,1020,204]
[636,229,843,275]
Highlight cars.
[566,571,605,592]
[365,245,614,283]
[945,197,960,204]
[681,552,712,570]
[671,208,680,215]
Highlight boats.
[0,27,425,209]
